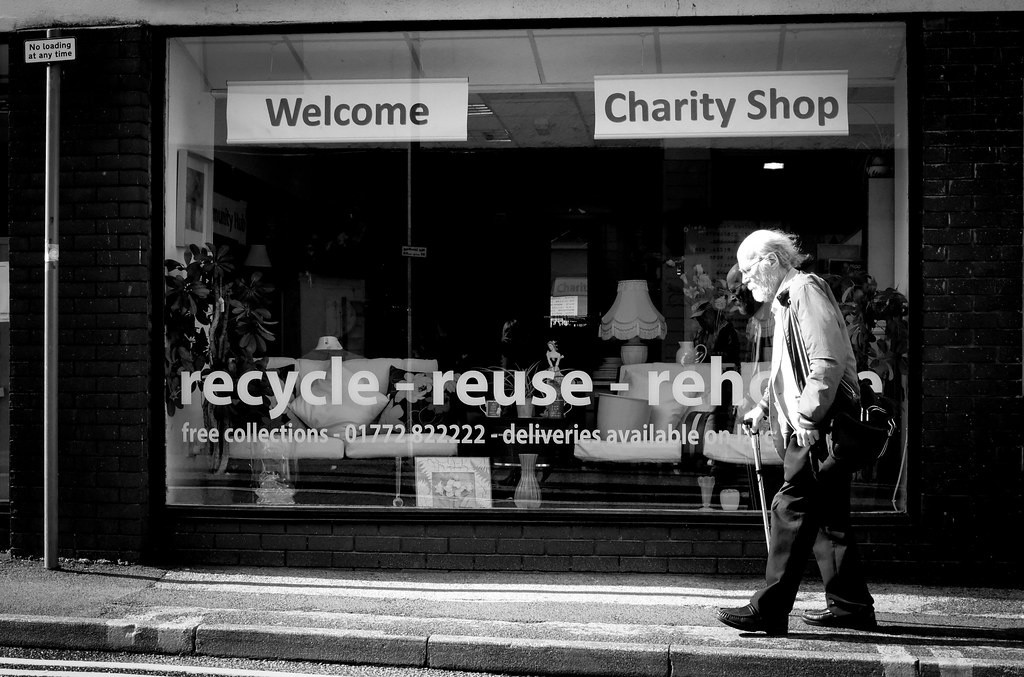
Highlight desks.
[475,410,576,483]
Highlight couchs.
[694,362,786,474]
[571,363,735,471]
[227,359,457,475]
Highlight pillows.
[378,366,472,433]
[290,366,389,440]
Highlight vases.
[598,393,652,441]
[514,454,541,509]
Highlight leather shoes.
[801,607,878,628]
[716,603,789,637]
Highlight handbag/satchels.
[806,380,898,485]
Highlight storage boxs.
[550,242,587,279]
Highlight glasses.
[728,283,745,296]
[738,257,766,278]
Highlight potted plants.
[851,107,895,177]
[476,361,543,418]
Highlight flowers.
[680,264,748,357]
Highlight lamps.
[597,279,668,365]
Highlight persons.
[727,263,785,513]
[715,229,878,635]
[546,341,560,382]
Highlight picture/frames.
[414,456,492,509]
[175,150,213,250]
